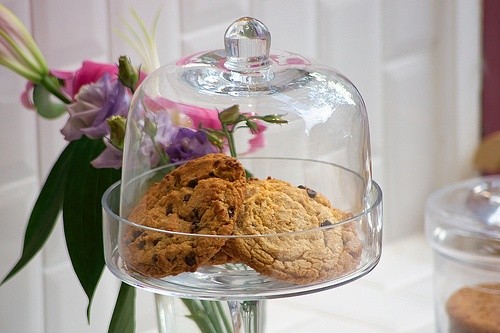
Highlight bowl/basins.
[428,174,500,333]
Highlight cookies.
[446,285,500,333]
[122,152,363,284]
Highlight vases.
[153,292,269,333]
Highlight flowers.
[1,2,258,332]
